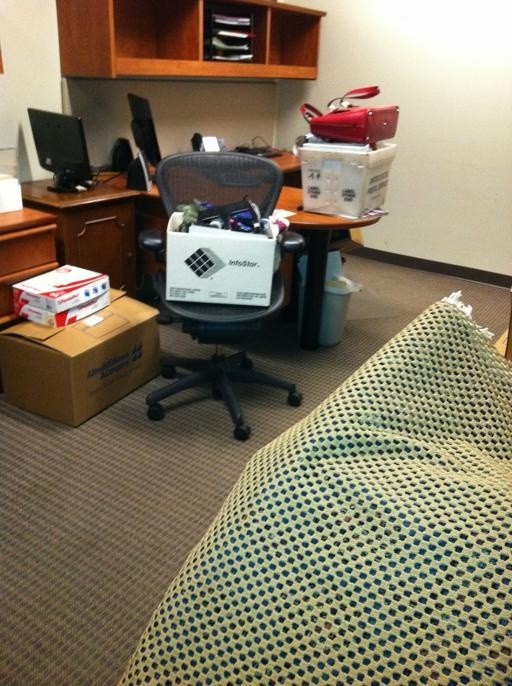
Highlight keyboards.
[227,147,282,158]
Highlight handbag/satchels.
[300,85,401,144]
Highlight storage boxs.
[11,262,110,315]
[0,283,164,428]
[11,289,110,327]
[291,135,398,217]
[163,209,277,306]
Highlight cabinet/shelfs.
[25,168,142,297]
[1,205,59,329]
[54,0,331,85]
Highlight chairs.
[144,149,304,441]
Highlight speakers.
[127,151,152,192]
[112,138,133,171]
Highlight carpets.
[2,250,512,685]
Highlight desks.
[140,144,384,352]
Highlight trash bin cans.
[297,276,354,348]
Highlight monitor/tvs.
[127,93,162,183]
[27,107,93,193]
